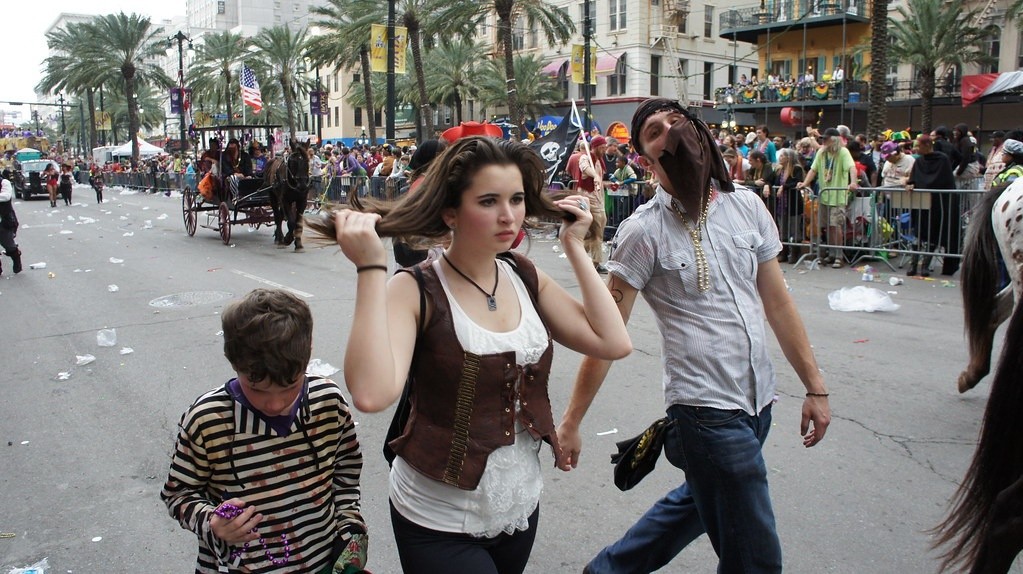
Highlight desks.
[819,4,840,16]
[753,13,772,24]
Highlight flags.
[239,62,262,115]
[183,89,193,127]
[961,71,1023,108]
[526,104,580,185]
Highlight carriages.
[179,124,321,255]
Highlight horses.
[263,139,312,253]
[923,175,1022,574]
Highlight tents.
[112,136,165,165]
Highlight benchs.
[193,160,263,205]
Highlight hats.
[409,146,418,150]
[1002,138,1023,155]
[818,127,840,139]
[382,143,393,152]
[912,133,933,147]
[123,160,132,167]
[744,131,757,144]
[879,140,898,158]
[589,134,607,148]
[988,131,1005,138]
[723,148,736,158]
[892,130,914,146]
[532,128,541,133]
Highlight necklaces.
[442,252,499,311]
[215,504,290,567]
[671,182,712,293]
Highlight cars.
[13,159,63,201]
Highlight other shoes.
[50,202,57,207]
[594,263,609,273]
[11,255,22,274]
[821,255,836,264]
[775,253,788,263]
[831,258,844,268]
[787,256,797,264]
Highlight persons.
[102,153,176,197]
[822,69,832,81]
[761,68,814,100]
[507,123,658,241]
[176,138,271,205]
[739,73,750,86]
[273,140,417,211]
[751,74,759,86]
[979,130,1023,188]
[551,98,832,574]
[708,123,978,277]
[0,120,103,276]
[832,65,844,99]
[295,134,633,574]
[159,288,372,574]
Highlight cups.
[553,246,558,252]
[889,277,903,286]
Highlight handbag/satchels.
[197,172,217,202]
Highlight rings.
[576,199,589,212]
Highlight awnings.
[595,52,626,77]
[540,60,567,78]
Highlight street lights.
[166,30,195,155]
[357,125,371,147]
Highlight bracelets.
[806,392,829,397]
[357,264,387,273]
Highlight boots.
[906,243,920,276]
[921,244,936,276]
